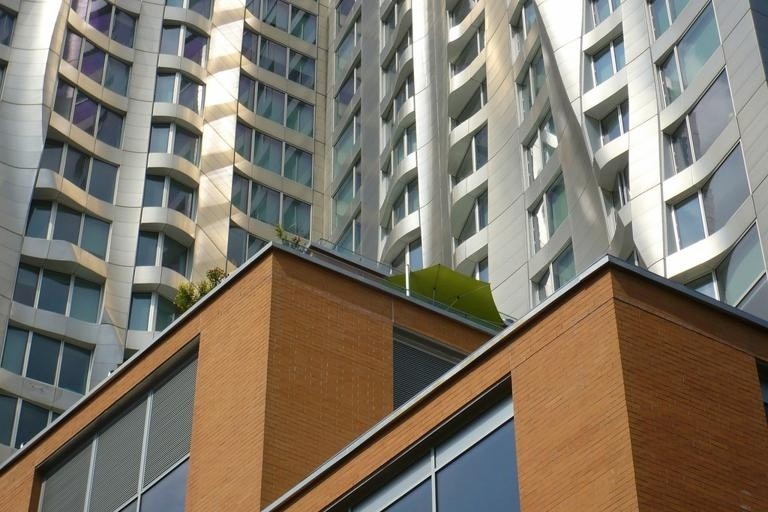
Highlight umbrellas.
[389,264,508,327]
